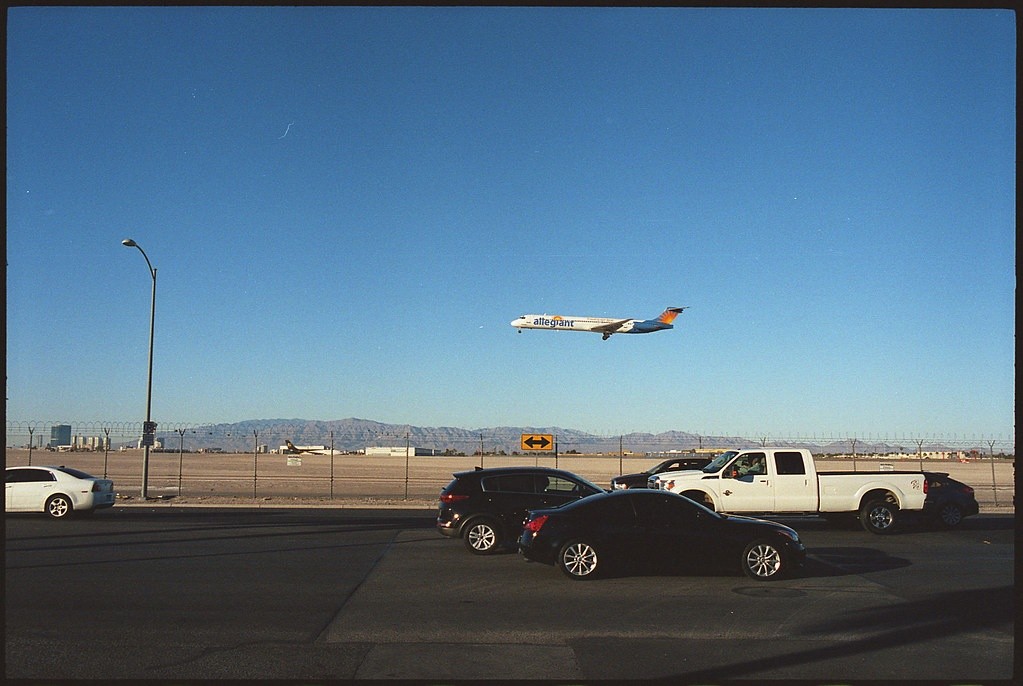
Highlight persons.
[739,456,767,476]
[535,476,550,492]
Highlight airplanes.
[510,306,691,341]
[286,440,344,458]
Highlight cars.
[5,466,115,521]
[436,466,606,556]
[609,458,713,490]
[517,490,807,582]
[820,472,979,530]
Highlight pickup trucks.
[647,447,928,534]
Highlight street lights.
[121,239,158,498]
